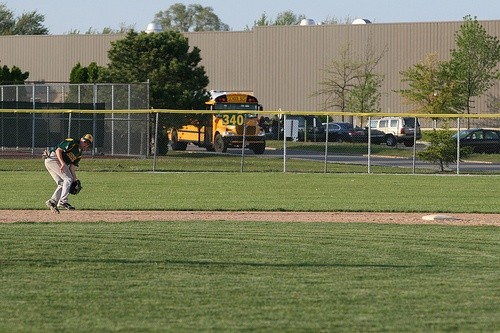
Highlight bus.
[162,89,267,153]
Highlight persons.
[46,134,94,214]
[259,115,280,139]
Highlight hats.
[82,134,95,145]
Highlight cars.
[451,128,500,155]
[276,115,328,140]
[321,121,385,145]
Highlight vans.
[365,116,422,147]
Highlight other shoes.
[45,199,60,215]
[57,202,76,211]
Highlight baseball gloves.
[70,180,82,195]
